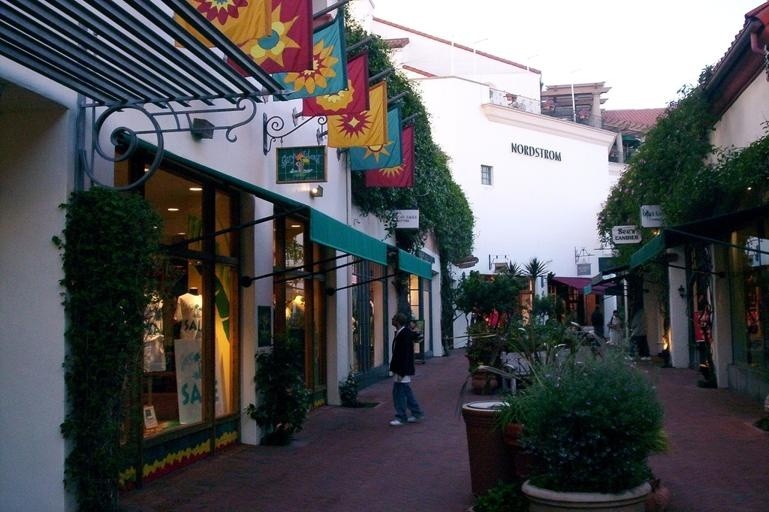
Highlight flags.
[171,1,271,45]
[226,0,313,77]
[274,10,347,101]
[302,54,369,116]
[327,81,387,148]
[365,126,414,188]
[349,104,402,169]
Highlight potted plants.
[501,348,667,512]
[472,373,498,395]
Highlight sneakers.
[390,417,425,427]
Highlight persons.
[390,313,425,426]
[289,295,305,313]
[591,305,604,348]
[607,310,624,349]
[172,287,202,339]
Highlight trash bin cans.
[461,400,514,499]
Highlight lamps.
[503,385,546,479]
[678,285,686,297]
[311,186,323,198]
[195,118,214,139]
